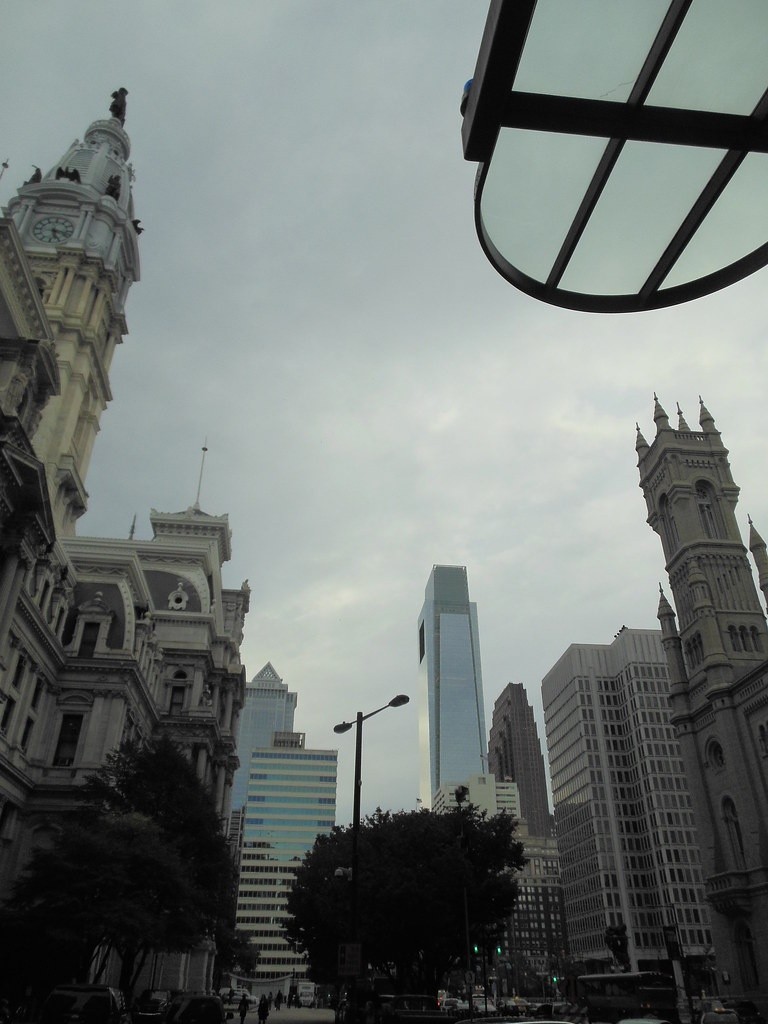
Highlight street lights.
[333,695,410,956]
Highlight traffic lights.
[497,945,502,954]
[473,944,478,954]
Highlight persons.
[226,988,321,1024]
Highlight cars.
[160,992,234,1024]
[32,983,133,1024]
[132,988,183,1024]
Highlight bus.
[576,970,677,1022]
